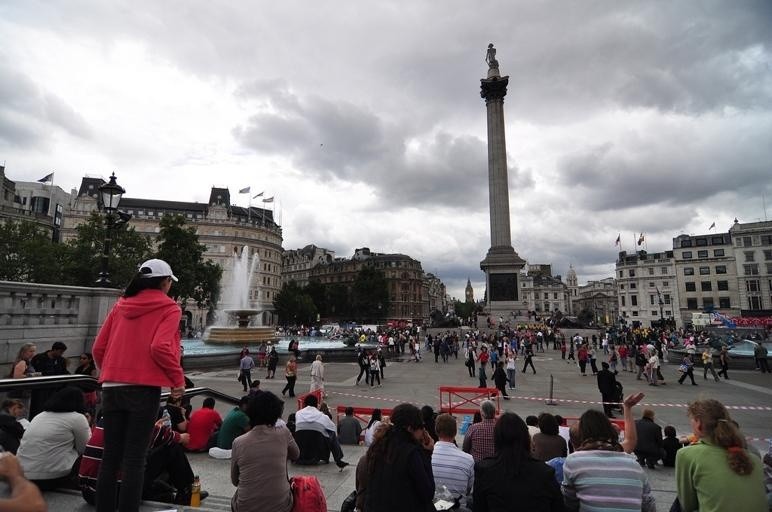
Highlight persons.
[486,43,497,67]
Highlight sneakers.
[243,375,296,397]
[635,372,728,387]
[337,462,350,468]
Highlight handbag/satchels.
[289,476,329,512]
[341,489,359,512]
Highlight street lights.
[658,299,665,330]
[594,303,600,324]
[94,171,126,287]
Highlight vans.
[318,322,385,335]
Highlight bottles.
[190,475,201,508]
[162,409,172,431]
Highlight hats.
[138,257,179,283]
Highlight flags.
[238,187,251,194]
[708,224,714,230]
[263,197,273,202]
[38,172,52,182]
[638,234,641,246]
[639,237,644,241]
[615,236,619,246]
[253,192,264,198]
